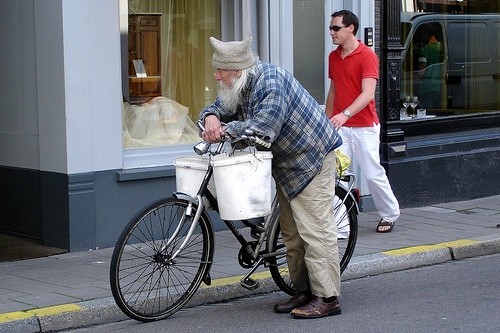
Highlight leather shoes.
[273,296,314,312]
[289,296,342,319]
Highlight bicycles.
[110,120,360,323]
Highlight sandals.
[376,218,395,233]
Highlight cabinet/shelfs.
[128,12,162,98]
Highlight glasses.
[329,26,348,32]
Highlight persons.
[198,35,343,319]
[325,9,401,240]
[413,25,446,111]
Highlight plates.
[413,115,436,118]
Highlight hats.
[208,35,255,70]
[418,57,426,63]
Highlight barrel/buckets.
[210,133,273,221]
[175,153,218,198]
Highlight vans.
[401,12,500,115]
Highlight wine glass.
[402,96,418,118]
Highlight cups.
[400,108,407,120]
[417,109,426,118]
